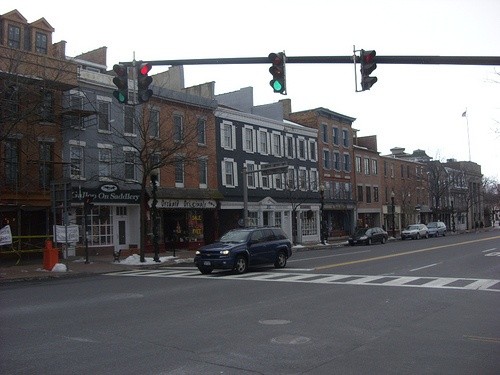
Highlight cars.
[347,226,389,245]
[401,223,430,240]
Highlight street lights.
[391,192,396,238]
[319,183,325,244]
[150,164,161,262]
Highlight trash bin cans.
[42,248,59,270]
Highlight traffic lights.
[268,51,287,95]
[138,60,154,104]
[359,49,378,90]
[112,64,128,102]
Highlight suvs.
[193,225,293,275]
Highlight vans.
[426,222,447,238]
